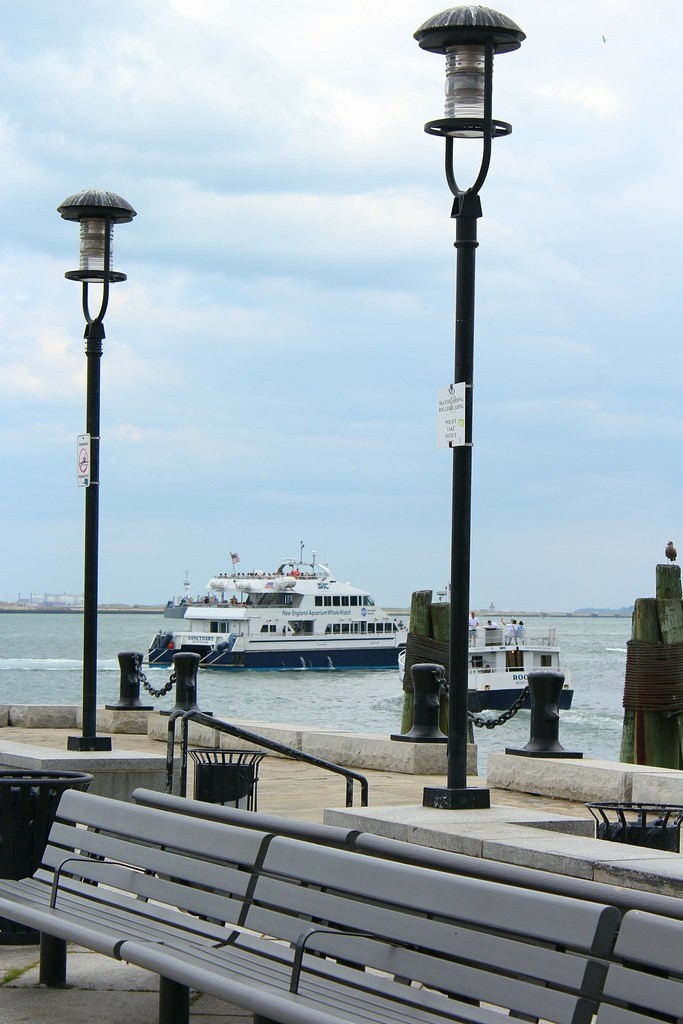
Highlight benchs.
[0,788,683,1024]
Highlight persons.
[469,612,480,647]
[487,620,499,627]
[293,623,301,635]
[179,594,238,607]
[289,568,300,580]
[393,618,404,630]
[500,618,526,645]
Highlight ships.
[144,544,404,672]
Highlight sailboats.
[394,606,575,713]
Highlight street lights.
[410,5,526,809]
[53,188,137,751]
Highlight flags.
[231,553,240,564]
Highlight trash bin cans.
[0,769,94,946]
[485,628,503,646]
[584,802,683,853]
[187,749,267,812]
